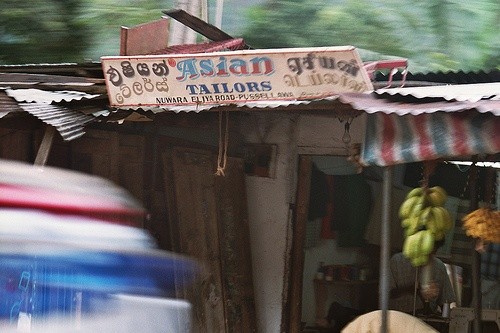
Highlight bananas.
[399,185,453,269]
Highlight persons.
[388,226,455,327]
[310,213,377,330]
